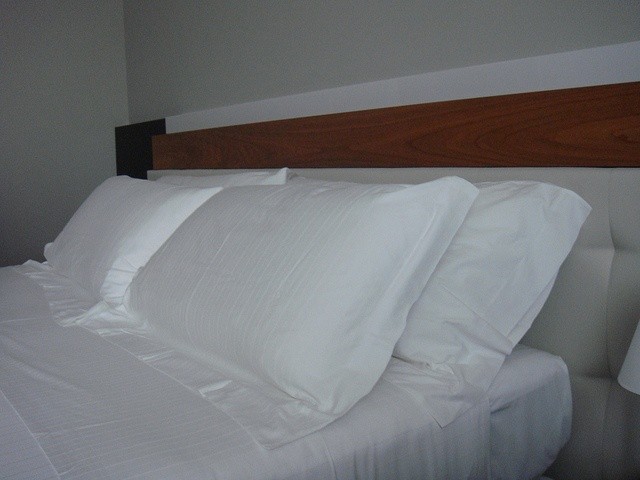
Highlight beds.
[0,38,640,480]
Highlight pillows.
[8,166,289,327]
[269,173,592,429]
[75,175,480,451]
